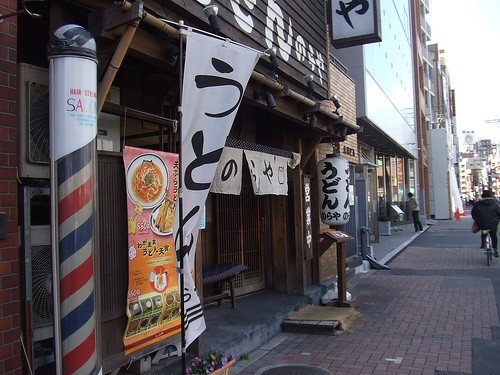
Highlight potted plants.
[377,216,392,236]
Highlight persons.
[407,192,423,233]
[471,191,500,258]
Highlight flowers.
[185,348,233,375]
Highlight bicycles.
[480,230,495,266]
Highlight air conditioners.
[19,62,120,370]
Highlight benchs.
[202,263,248,308]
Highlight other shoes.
[420,229,423,231]
[494,250,498,258]
[415,229,418,231]
[481,241,487,248]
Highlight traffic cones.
[455,208,461,221]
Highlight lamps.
[340,126,348,137]
[265,47,279,76]
[304,74,315,100]
[203,4,221,32]
[255,86,276,108]
[330,94,341,115]
[303,112,317,129]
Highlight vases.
[191,359,236,375]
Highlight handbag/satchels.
[472,223,480,233]
